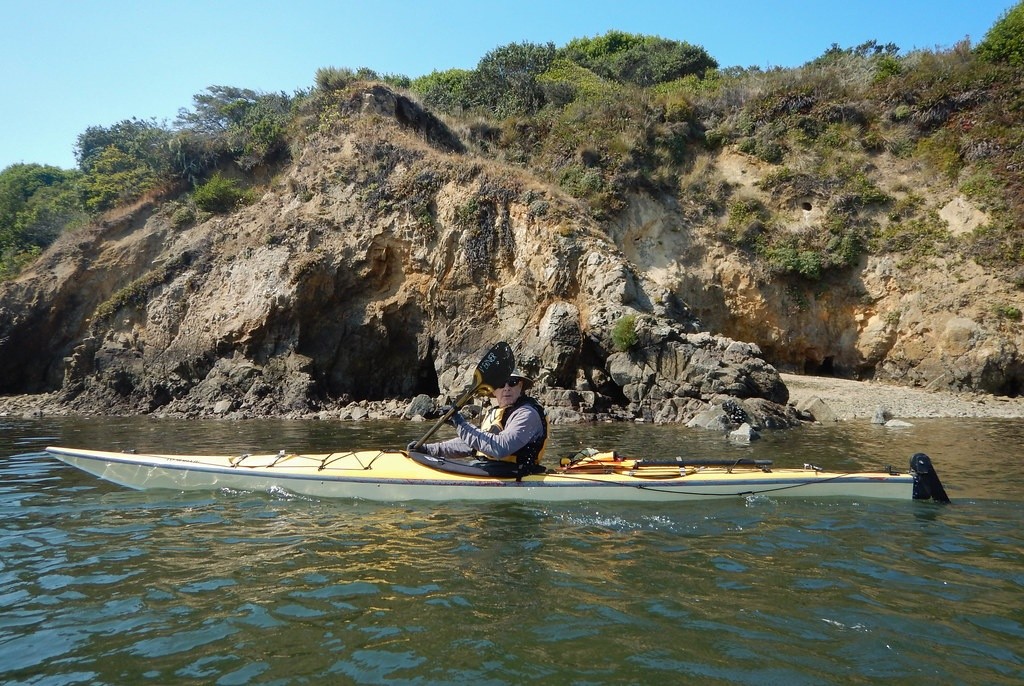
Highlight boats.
[42,444,952,500]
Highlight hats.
[510,367,534,389]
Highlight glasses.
[494,377,524,388]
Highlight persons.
[407,363,550,470]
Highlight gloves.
[407,441,428,454]
[438,406,463,421]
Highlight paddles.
[416,341,515,450]
